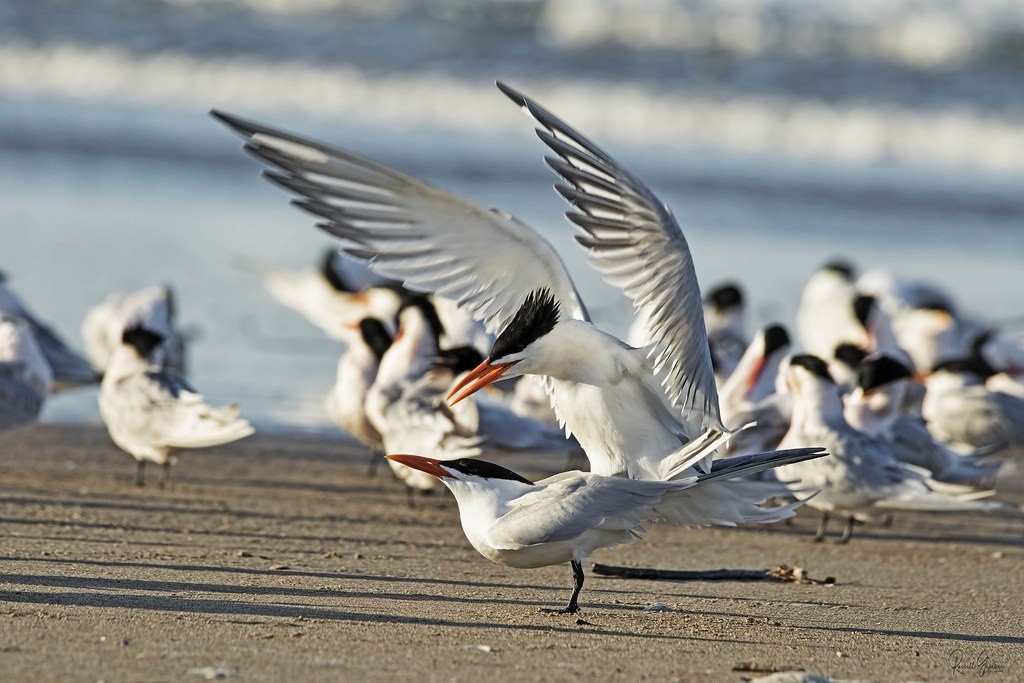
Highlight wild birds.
[768,353,1020,547]
[0,270,193,430]
[208,78,823,528]
[277,252,1024,511]
[383,418,833,616]
[98,324,257,496]
